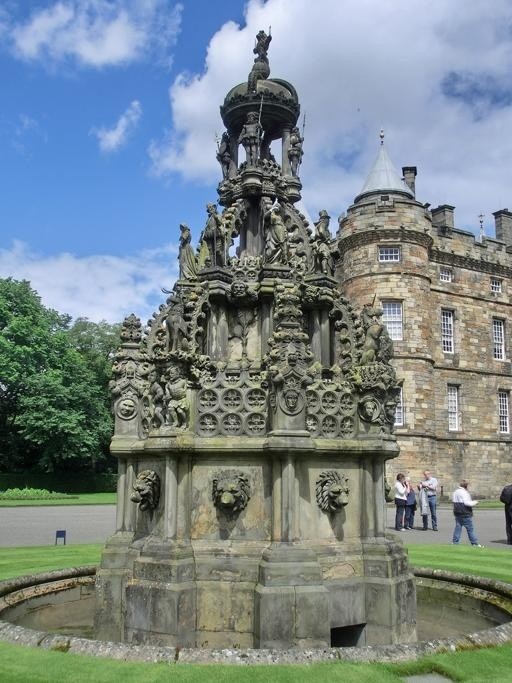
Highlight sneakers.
[473,543,487,548]
[395,526,438,531]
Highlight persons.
[452,479,485,547]
[393,473,408,532]
[101,26,401,439]
[499,483,511,544]
[416,470,438,531]
[403,476,417,530]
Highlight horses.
[360,302,385,329]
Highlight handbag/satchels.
[388,487,398,500]
